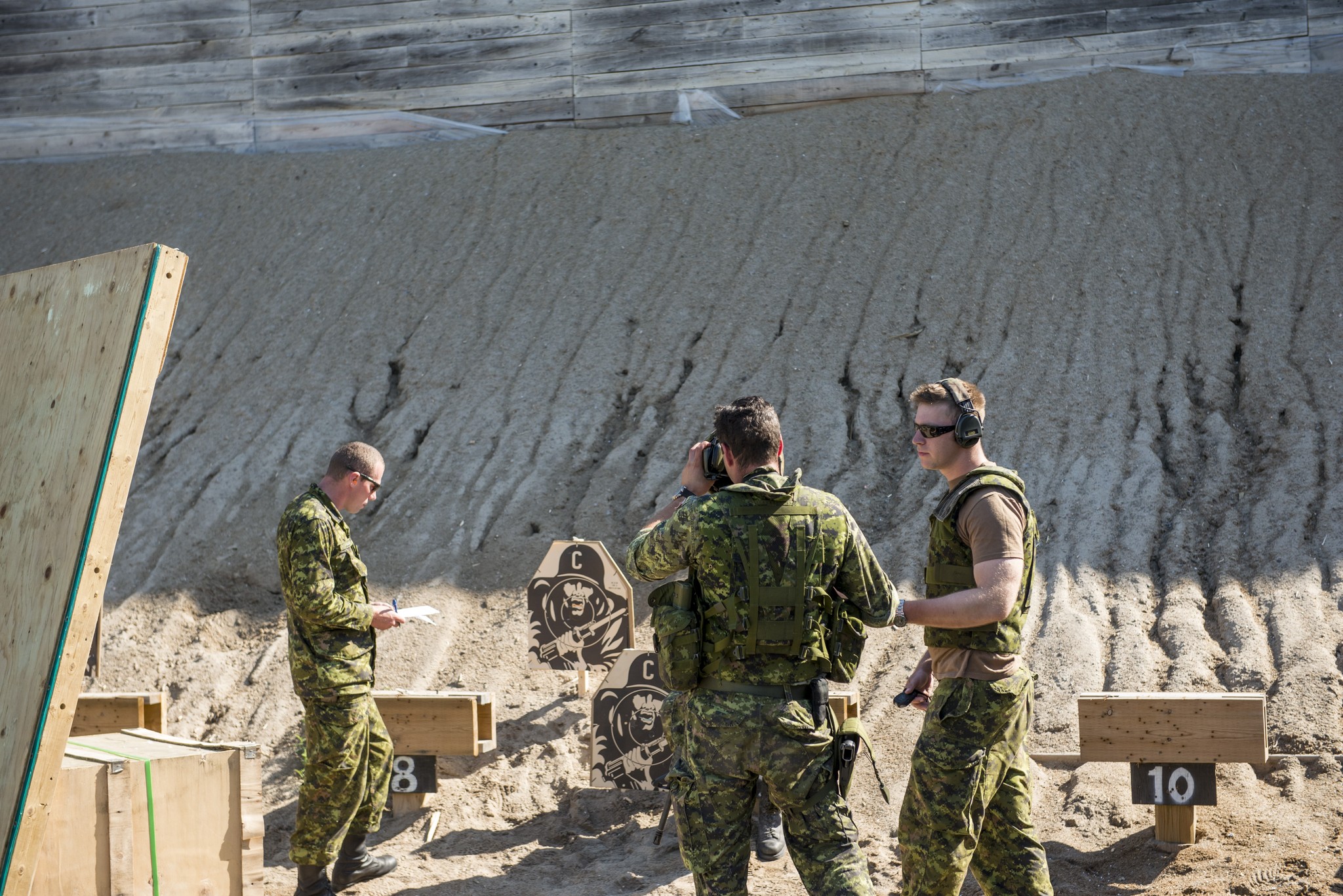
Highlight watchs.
[672,486,696,500]
[894,598,907,628]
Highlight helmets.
[699,427,785,493]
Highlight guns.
[839,733,857,783]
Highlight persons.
[273,441,411,896]
[877,371,1058,896]
[747,775,785,861]
[625,393,899,896]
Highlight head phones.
[702,435,733,484]
[936,377,984,449]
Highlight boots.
[755,777,787,862]
[292,864,335,896]
[332,830,397,892]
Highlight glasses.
[344,464,382,492]
[913,420,961,439]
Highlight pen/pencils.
[392,599,401,628]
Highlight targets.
[588,648,675,794]
[525,536,637,700]
